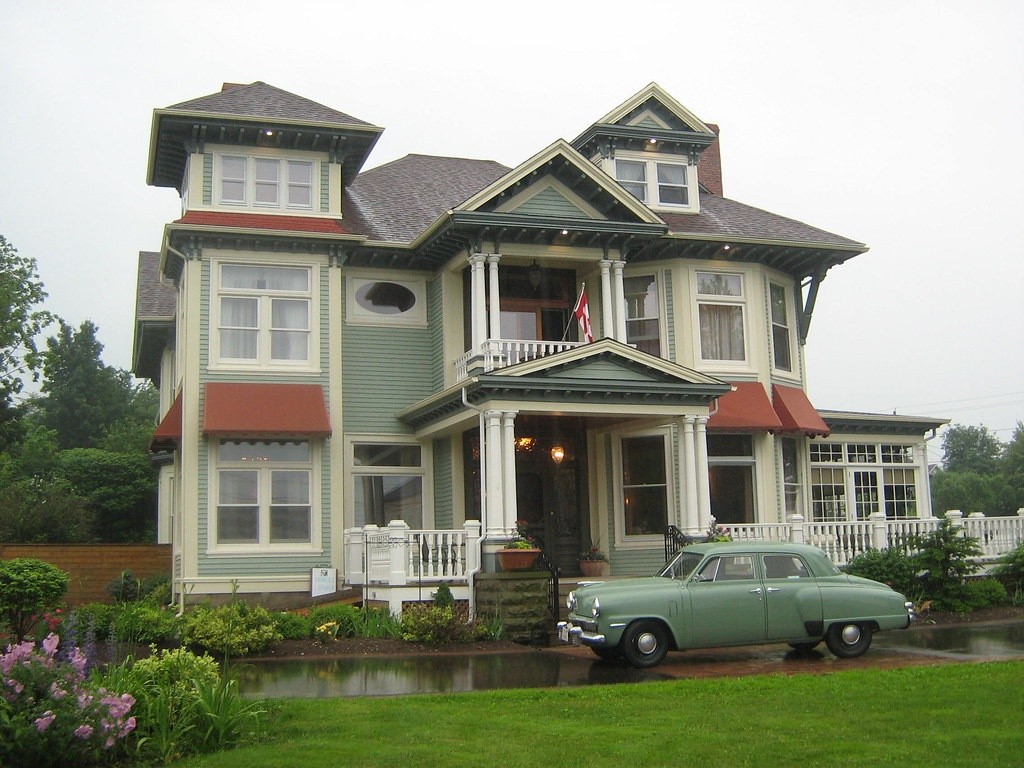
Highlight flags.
[575,292,594,343]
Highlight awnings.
[705,381,783,435]
[149,387,183,453]
[773,384,831,439]
[203,382,332,447]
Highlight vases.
[578,559,604,577]
[496,545,540,569]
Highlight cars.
[557,540,914,666]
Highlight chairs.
[416,534,458,575]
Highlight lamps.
[550,445,565,464]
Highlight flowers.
[577,540,614,562]
[503,520,544,549]
